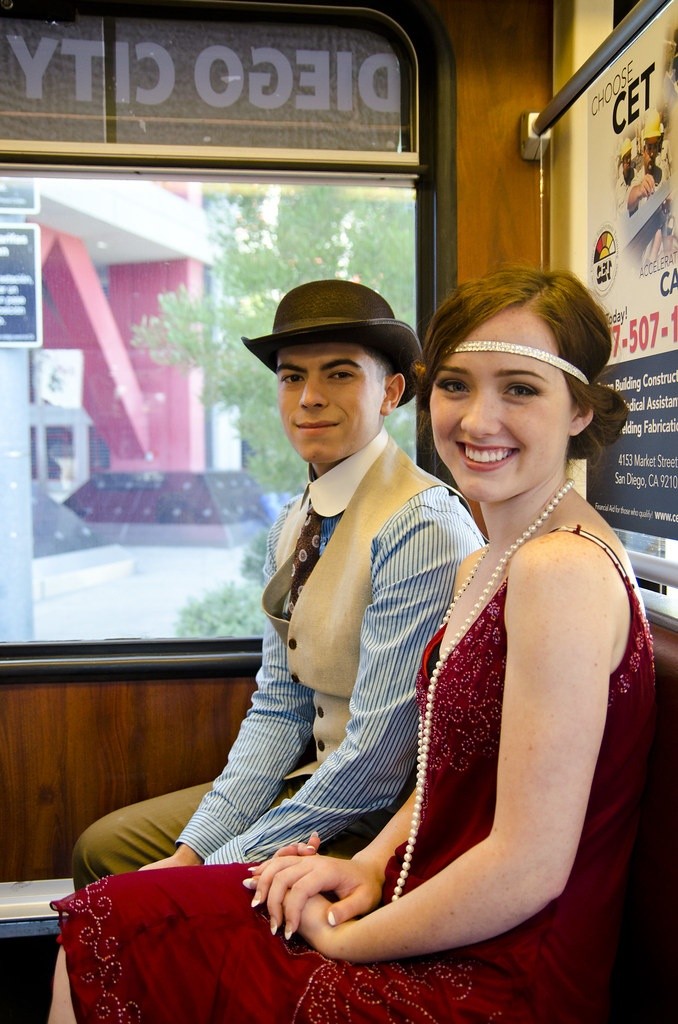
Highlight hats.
[241,280,422,408]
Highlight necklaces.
[389,475,576,902]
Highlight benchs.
[605,550,678,1024]
[0,877,75,939]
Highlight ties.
[286,497,325,623]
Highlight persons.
[47,266,656,1024]
[614,108,672,262]
